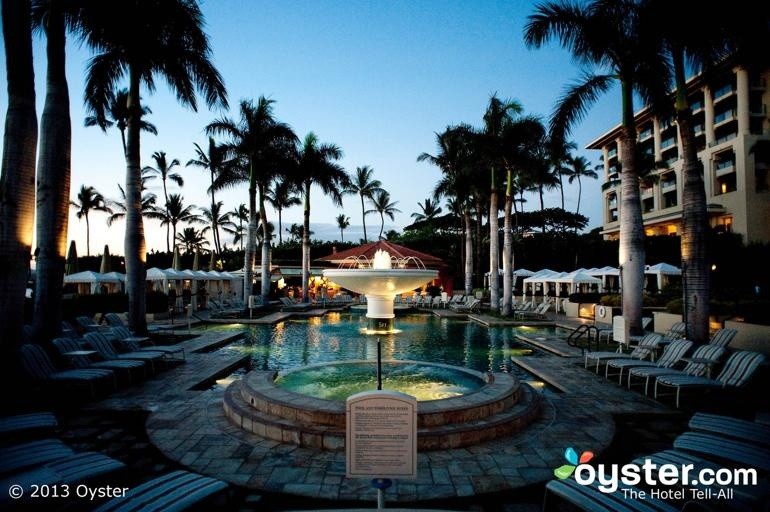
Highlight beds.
[99,243,112,273]
[190,244,201,313]
[205,249,222,298]
[172,245,185,314]
[65,238,79,276]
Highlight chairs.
[395,291,552,321]
[543,317,769,511]
[281,292,353,312]
[0,315,232,511]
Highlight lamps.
[395,291,552,321]
[281,292,353,312]
